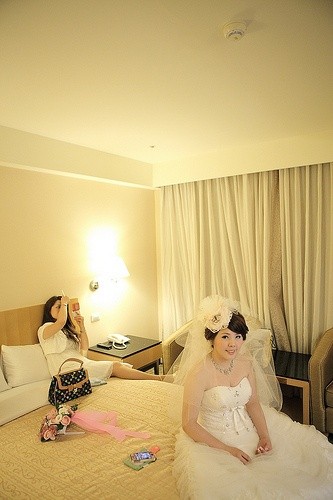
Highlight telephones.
[107,333,130,344]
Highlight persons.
[37,296,178,387]
[160,294,333,500]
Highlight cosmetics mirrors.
[72,302,80,318]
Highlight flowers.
[40,403,79,441]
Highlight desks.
[271,348,311,425]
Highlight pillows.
[1,343,53,391]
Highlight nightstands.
[86,335,162,376]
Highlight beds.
[0,298,290,500]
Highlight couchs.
[308,328,333,437]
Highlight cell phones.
[130,452,152,461]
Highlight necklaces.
[211,351,234,375]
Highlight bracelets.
[80,329,85,333]
[61,304,67,307]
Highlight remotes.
[97,343,112,350]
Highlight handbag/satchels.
[49,358,91,406]
